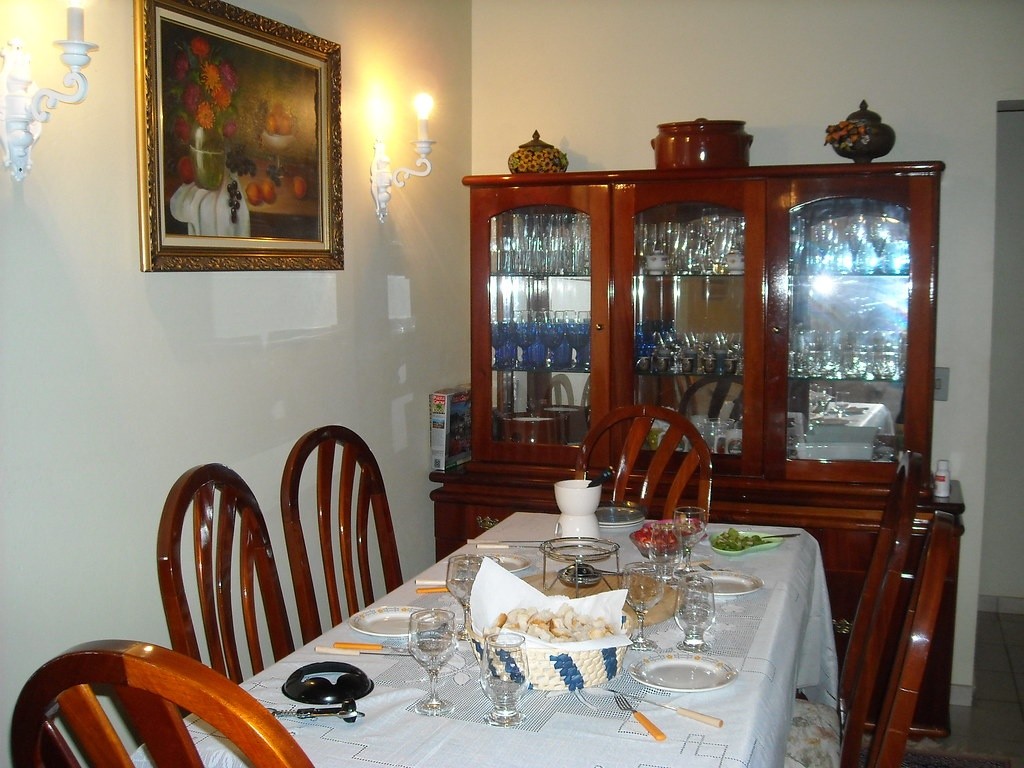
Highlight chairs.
[283,426,402,639]
[8,640,316,768]
[870,510,954,766]
[158,464,298,688]
[835,449,923,767]
[573,405,714,522]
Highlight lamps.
[0,0,102,182]
[365,81,437,222]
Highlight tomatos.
[633,525,682,556]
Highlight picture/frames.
[136,0,341,272]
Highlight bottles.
[934,460,954,498]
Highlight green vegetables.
[713,528,772,552]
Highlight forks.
[333,643,409,654]
[613,694,666,741]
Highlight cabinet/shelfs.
[432,160,967,738]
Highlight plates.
[591,506,647,527]
[683,571,764,596]
[708,531,783,555]
[815,417,849,427]
[460,552,532,574]
[627,648,738,693]
[349,606,443,637]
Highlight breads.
[489,604,617,641]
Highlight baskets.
[464,610,639,690]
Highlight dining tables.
[130,510,839,768]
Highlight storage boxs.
[430,383,471,472]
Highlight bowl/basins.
[629,529,702,560]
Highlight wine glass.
[446,555,484,642]
[622,563,665,650]
[480,633,531,727]
[673,575,716,653]
[486,208,914,453]
[671,507,707,575]
[408,609,455,716]
